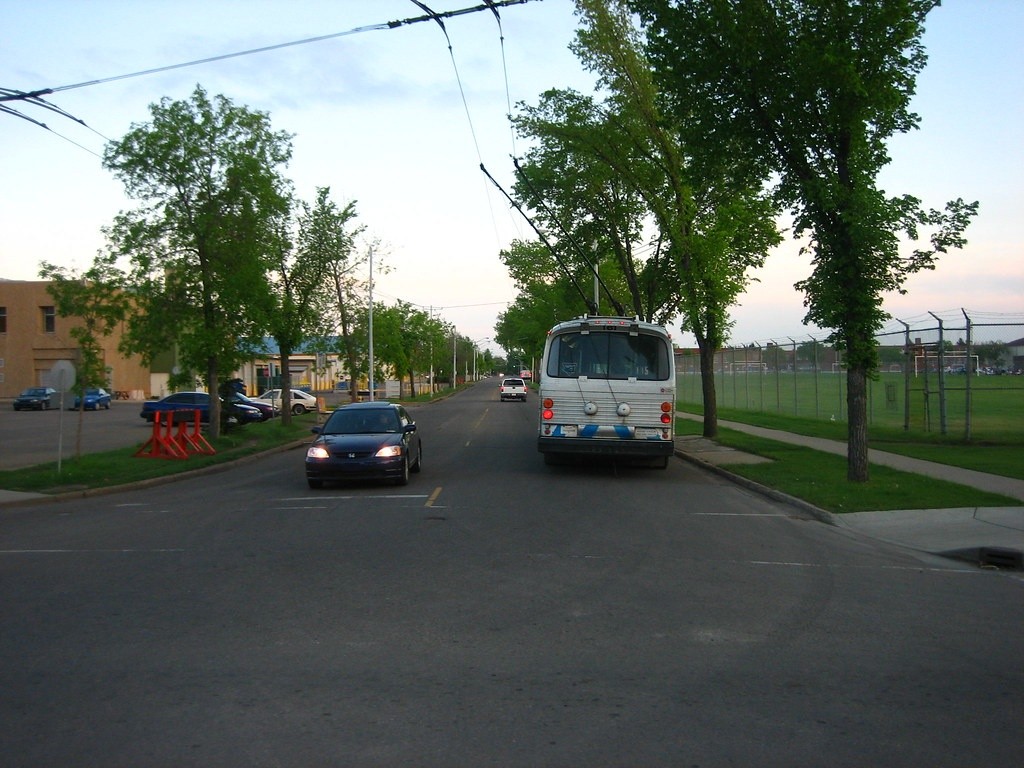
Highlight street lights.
[472,337,491,382]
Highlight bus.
[537,312,677,472]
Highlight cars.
[498,378,528,403]
[12,386,60,411]
[304,401,424,490]
[72,386,111,411]
[219,390,279,422]
[247,389,317,415]
[139,391,263,427]
